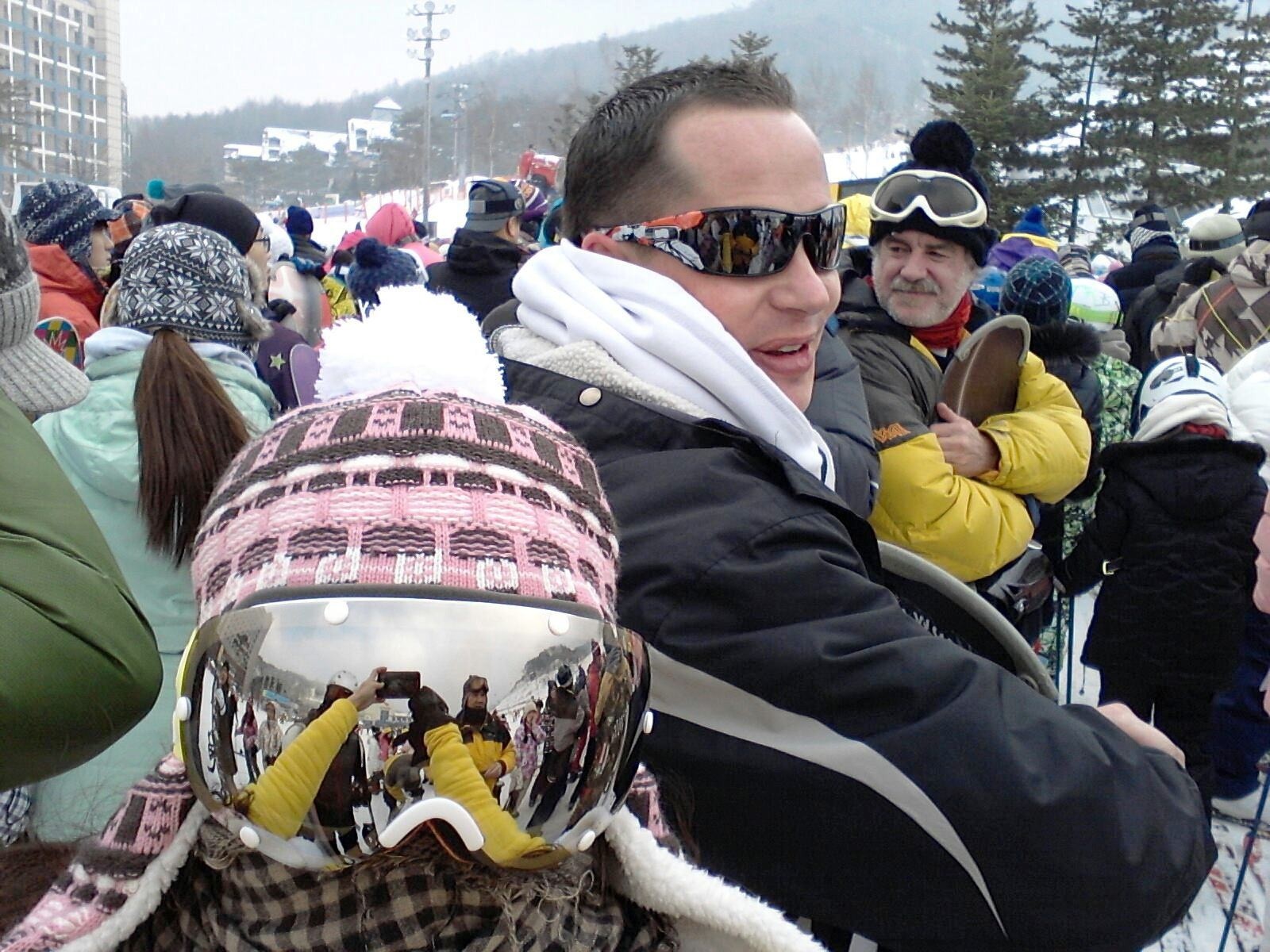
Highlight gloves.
[277,252,326,281]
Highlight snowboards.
[289,344,324,411]
[36,317,85,376]
[938,316,1032,429]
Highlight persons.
[28,221,284,840]
[0,289,830,952]
[258,701,282,771]
[236,697,261,782]
[234,666,553,862]
[1055,353,1268,839]
[831,118,1090,641]
[509,700,546,817]
[525,621,635,840]
[455,675,518,795]
[215,660,238,799]
[381,753,433,826]
[363,724,439,796]
[280,684,371,861]
[0,152,1270,798]
[487,56,1220,952]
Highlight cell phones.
[377,671,421,698]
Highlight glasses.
[178,586,651,869]
[250,229,272,256]
[466,676,489,695]
[582,202,849,278]
[867,169,991,231]
[398,247,429,287]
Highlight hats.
[111,197,152,251]
[865,119,998,266]
[256,213,295,263]
[509,179,547,219]
[462,673,488,710]
[0,200,92,414]
[346,241,423,309]
[117,223,265,363]
[17,180,122,295]
[465,181,526,233]
[522,701,536,722]
[150,191,260,258]
[589,638,601,659]
[147,181,224,201]
[978,194,1270,441]
[0,286,826,952]
[557,664,572,689]
[286,206,313,234]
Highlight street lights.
[404,2,458,231]
[450,82,468,198]
